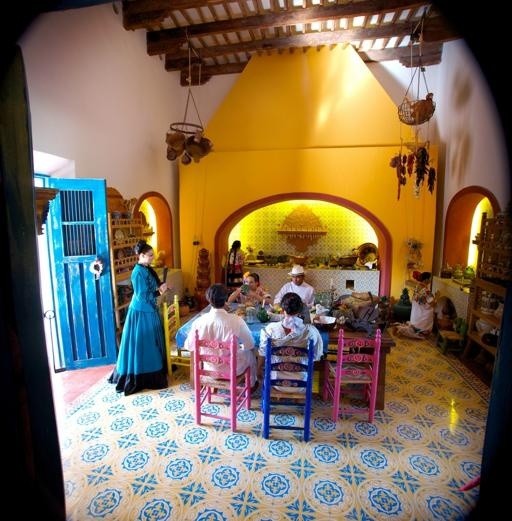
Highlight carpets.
[58,321,491,520]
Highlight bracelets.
[158,288,163,296]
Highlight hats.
[287,266,305,275]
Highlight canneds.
[246,306,256,324]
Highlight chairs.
[320,325,384,422]
[189,328,253,432]
[162,293,193,377]
[436,316,470,355]
[261,336,314,443]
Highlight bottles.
[315,289,322,302]
[182,287,192,308]
[122,212,131,225]
[452,318,467,336]
[112,211,122,225]
[239,283,250,296]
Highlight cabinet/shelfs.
[107,210,147,333]
[458,210,512,375]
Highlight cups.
[246,307,255,324]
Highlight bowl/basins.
[267,306,285,320]
[292,256,308,265]
[338,256,357,268]
[354,243,378,267]
[313,315,337,331]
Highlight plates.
[244,260,265,264]
[117,250,128,260]
[113,229,127,245]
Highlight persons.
[392,272,440,341]
[185,241,316,406]
[107,240,175,397]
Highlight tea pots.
[257,308,271,324]
